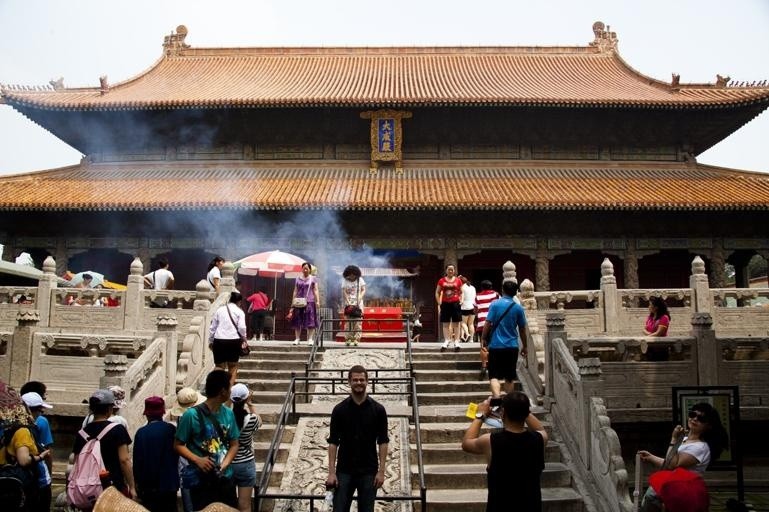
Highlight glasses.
[688,412,706,422]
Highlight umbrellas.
[233,250,318,339]
[70,270,104,288]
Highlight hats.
[20,382,250,417]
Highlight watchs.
[474,411,485,420]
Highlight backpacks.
[66,422,120,508]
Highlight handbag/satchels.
[345,305,361,317]
[641,486,663,512]
[319,484,335,512]
[240,342,249,357]
[483,327,493,347]
[292,298,307,308]
[0,454,41,512]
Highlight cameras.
[490,398,502,407]
[208,457,223,479]
[38,442,49,453]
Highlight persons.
[638,402,729,487]
[412,315,423,342]
[143,257,175,308]
[483,281,527,410]
[206,255,271,341]
[643,296,671,361]
[93,284,109,306]
[0,370,261,511]
[462,391,548,512]
[436,265,500,381]
[76,274,93,307]
[325,364,388,511]
[341,265,366,346]
[208,292,248,406]
[290,263,320,346]
